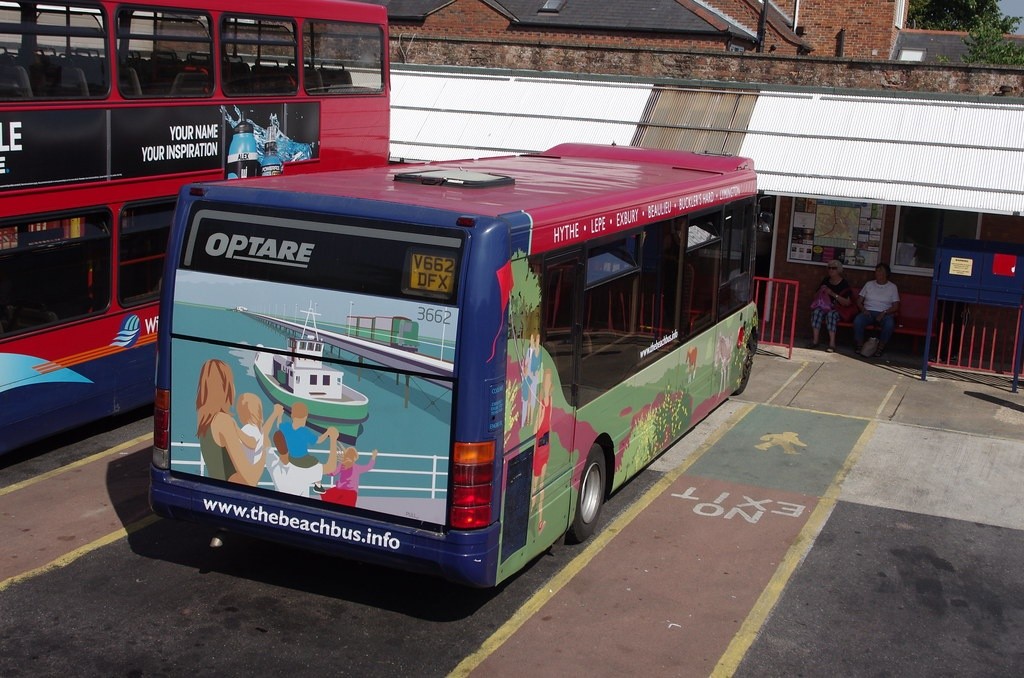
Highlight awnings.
[338,62,1024,216]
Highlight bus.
[0,0,390,455]
[145,141,774,590]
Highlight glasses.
[829,266,838,271]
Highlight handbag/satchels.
[810,288,832,313]
[861,338,879,358]
[835,304,858,322]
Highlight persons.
[852,263,900,366]
[808,259,849,352]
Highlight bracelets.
[836,294,839,301]
[884,310,887,315]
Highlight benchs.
[837,289,938,338]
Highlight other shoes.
[875,348,882,357]
[827,346,837,352]
[856,345,861,354]
[807,342,819,349]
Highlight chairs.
[0,47,352,100]
